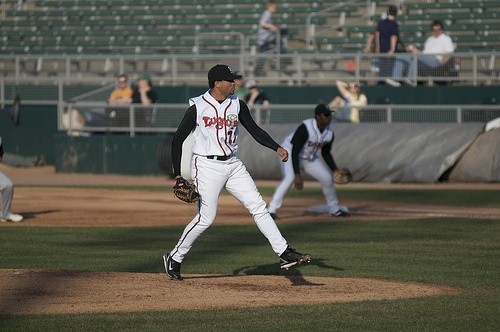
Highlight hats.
[233,71,243,80]
[315,103,335,114]
[245,80,257,89]
[208,64,242,81]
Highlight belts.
[207,154,234,160]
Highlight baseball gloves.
[332,167,352,184]
[173,179,201,203]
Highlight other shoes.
[270,213,276,220]
[1,212,24,222]
[332,210,350,217]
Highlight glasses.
[324,112,331,116]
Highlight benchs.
[0,0,500,53]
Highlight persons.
[364,29,381,71]
[61,101,86,136]
[231,71,248,104]
[374,5,399,86]
[244,80,270,124]
[162,64,313,281]
[267,104,352,219]
[130,72,156,134]
[327,79,367,122]
[0,136,24,223]
[108,75,134,134]
[251,0,288,84]
[386,21,456,87]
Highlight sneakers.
[280,246,312,270]
[162,252,183,281]
